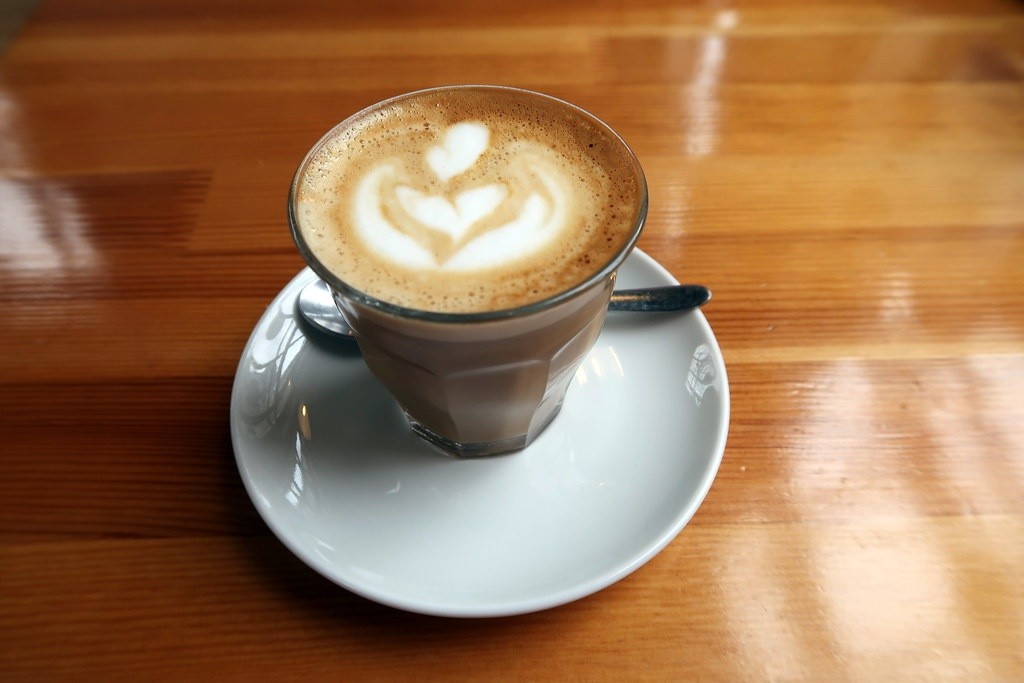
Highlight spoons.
[298,276,712,337]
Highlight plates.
[229,245,730,618]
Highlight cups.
[288,85,650,459]
